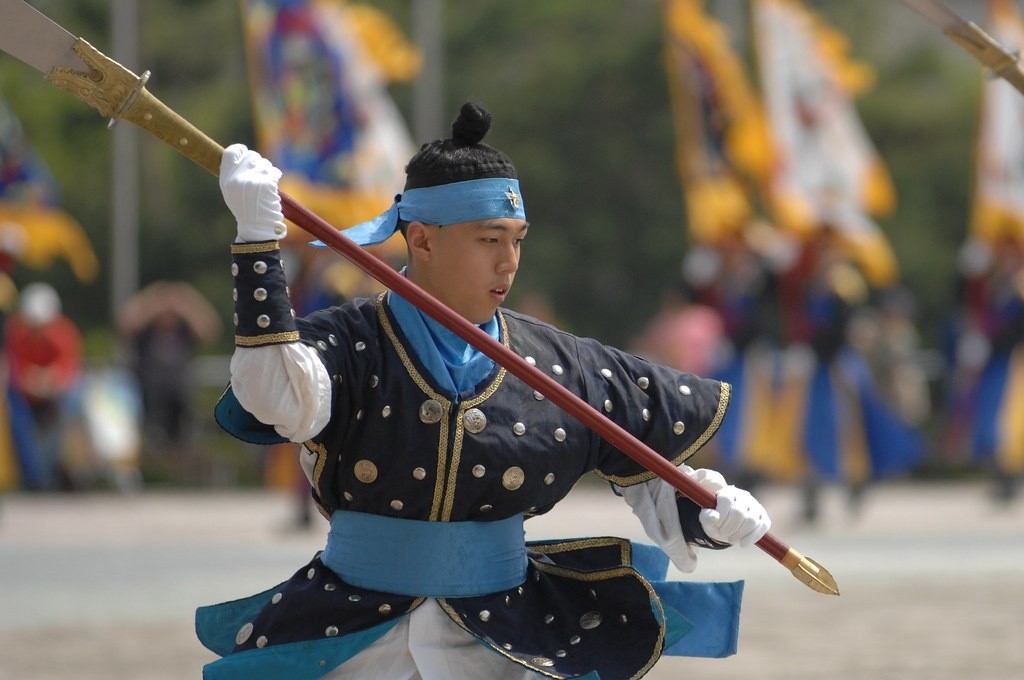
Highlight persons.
[194,101,770,680]
[678,214,1023,520]
[0,252,350,529]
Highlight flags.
[750,0,899,288]
[0,104,99,280]
[664,0,776,240]
[971,0,1024,231]
[240,0,425,256]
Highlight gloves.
[699,485,772,550]
[220,143,287,241]
